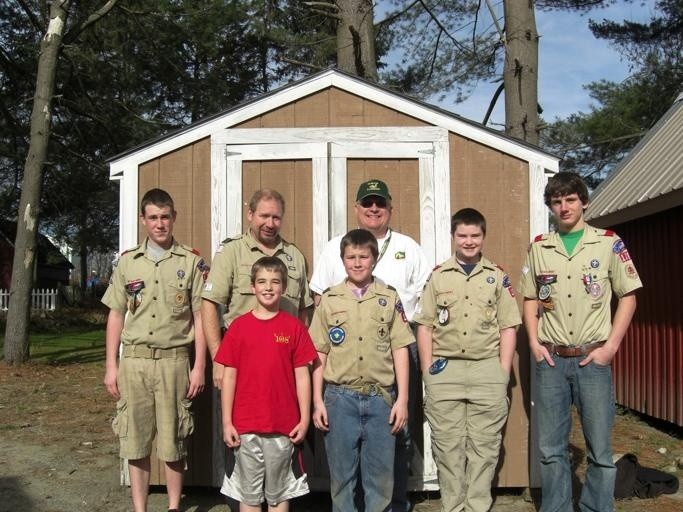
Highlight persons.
[101,187,210,512]
[202,189,314,359]
[411,207,522,512]
[308,228,418,512]
[215,257,320,512]
[515,172,643,512]
[308,178,433,512]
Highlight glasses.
[358,199,387,209]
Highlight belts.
[120,343,190,361]
[541,339,608,358]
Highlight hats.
[354,179,392,201]
